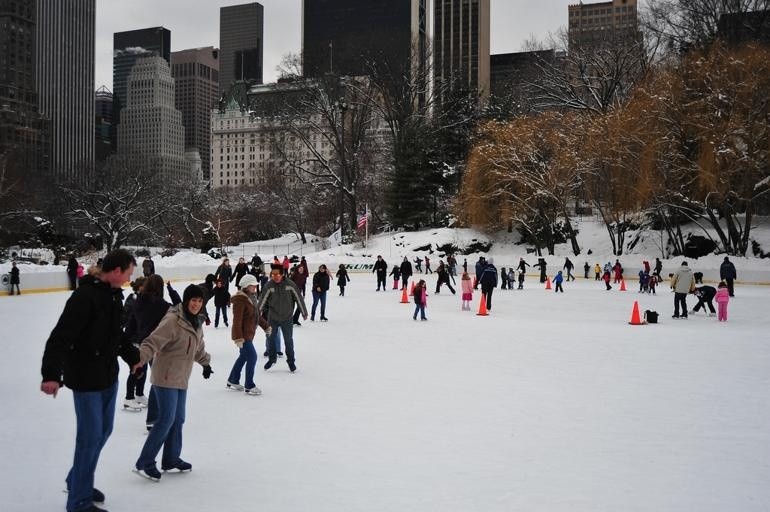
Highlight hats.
[237,273,263,288]
[182,283,203,309]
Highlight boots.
[287,358,296,371]
[134,453,161,479]
[263,358,277,371]
[159,458,193,471]
[227,379,244,391]
[123,392,150,410]
[65,481,105,504]
[243,381,259,394]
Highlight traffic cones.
[409,281,416,297]
[542,276,554,291]
[475,293,491,317]
[399,287,410,304]
[619,279,628,293]
[629,298,646,326]
[472,276,479,290]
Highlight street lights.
[332,95,357,239]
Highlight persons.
[214,253,350,395]
[373,255,498,321]
[124,260,213,481]
[584,259,624,290]
[67,255,103,290]
[41,249,144,512]
[638,258,663,293]
[501,257,531,289]
[8,262,21,295]
[533,257,575,292]
[669,256,736,321]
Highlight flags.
[358,213,366,228]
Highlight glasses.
[269,271,282,278]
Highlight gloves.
[201,362,214,379]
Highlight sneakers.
[292,315,329,327]
